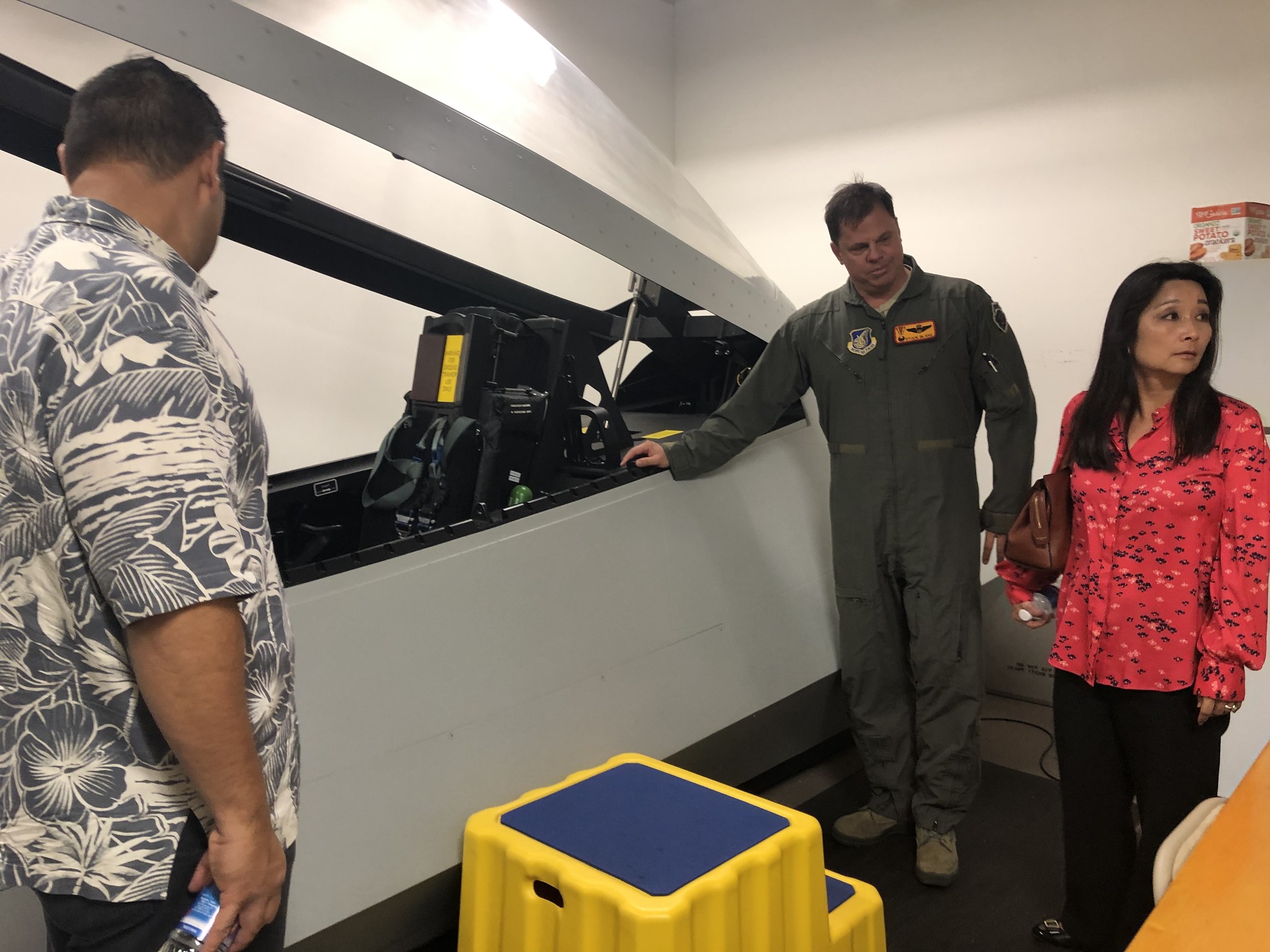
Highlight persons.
[994,259,1270,952]
[622,182,1038,890]
[0,55,300,952]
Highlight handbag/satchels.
[1003,466,1071,571]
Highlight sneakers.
[915,822,960,886]
[832,805,907,848]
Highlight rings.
[1224,705,1232,713]
[1232,705,1238,713]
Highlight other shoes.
[1033,916,1080,947]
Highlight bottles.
[158,881,240,952]
[1018,574,1061,622]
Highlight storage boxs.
[1188,201,1270,261]
